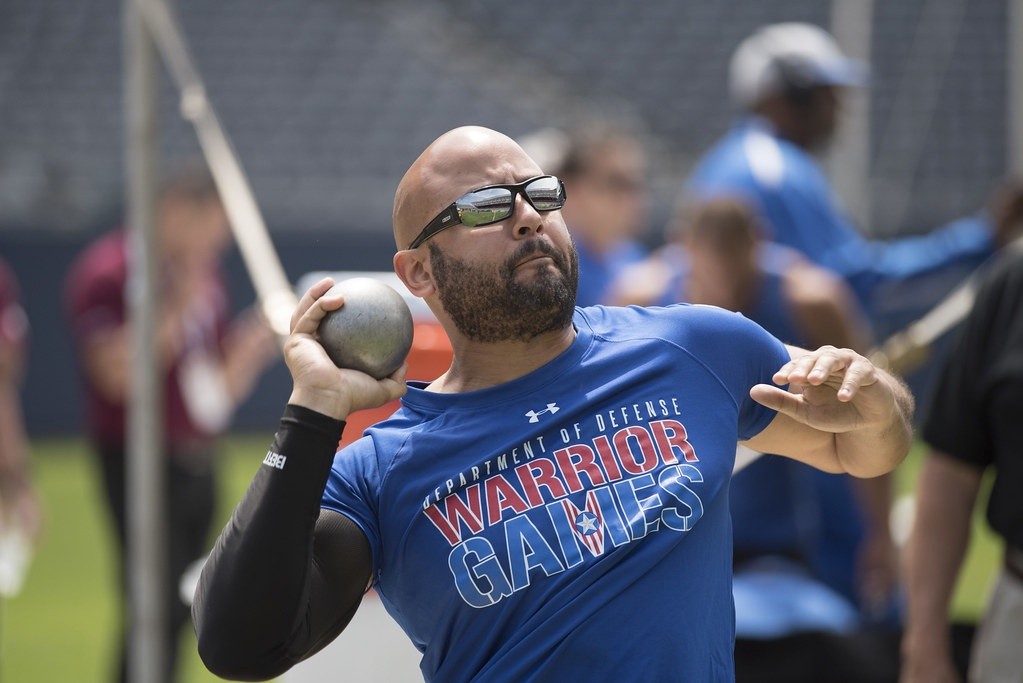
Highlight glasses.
[408,175,567,250]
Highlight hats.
[728,21,869,105]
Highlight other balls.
[315,278,415,382]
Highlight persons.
[668,23,1023,335]
[67,168,280,682]
[0,256,40,540]
[192,126,913,683]
[458,208,464,220]
[600,195,897,641]
[531,106,656,306]
[901,238,1023,683]
[469,208,479,214]
